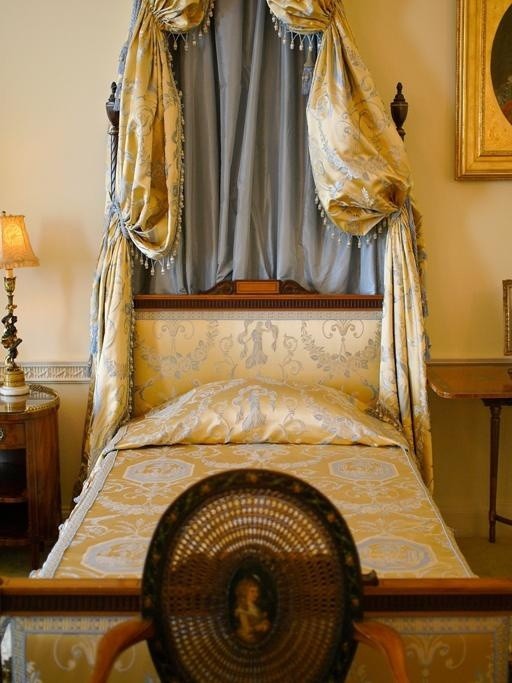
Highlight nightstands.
[0,383,60,569]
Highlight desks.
[424,359,511,545]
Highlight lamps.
[0,210,40,397]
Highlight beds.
[0,278,511,681]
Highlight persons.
[233,577,270,646]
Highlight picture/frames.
[456,0,512,181]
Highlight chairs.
[90,464,410,682]
